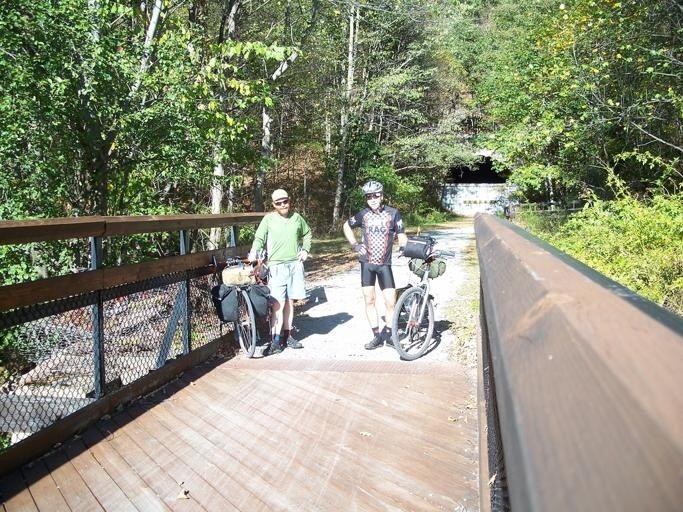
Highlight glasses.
[275,200,288,206]
[367,193,381,199]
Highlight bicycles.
[390,235,455,360]
[207,248,271,358]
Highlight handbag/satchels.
[221,265,257,286]
[409,257,445,278]
[406,237,434,258]
[247,286,271,318]
[212,285,238,322]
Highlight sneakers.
[365,336,383,350]
[284,338,302,348]
[386,339,394,346]
[264,341,282,356]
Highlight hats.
[272,189,288,200]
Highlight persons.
[342,180,408,350]
[248,188,312,356]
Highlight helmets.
[362,181,383,194]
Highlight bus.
[396,136,559,219]
